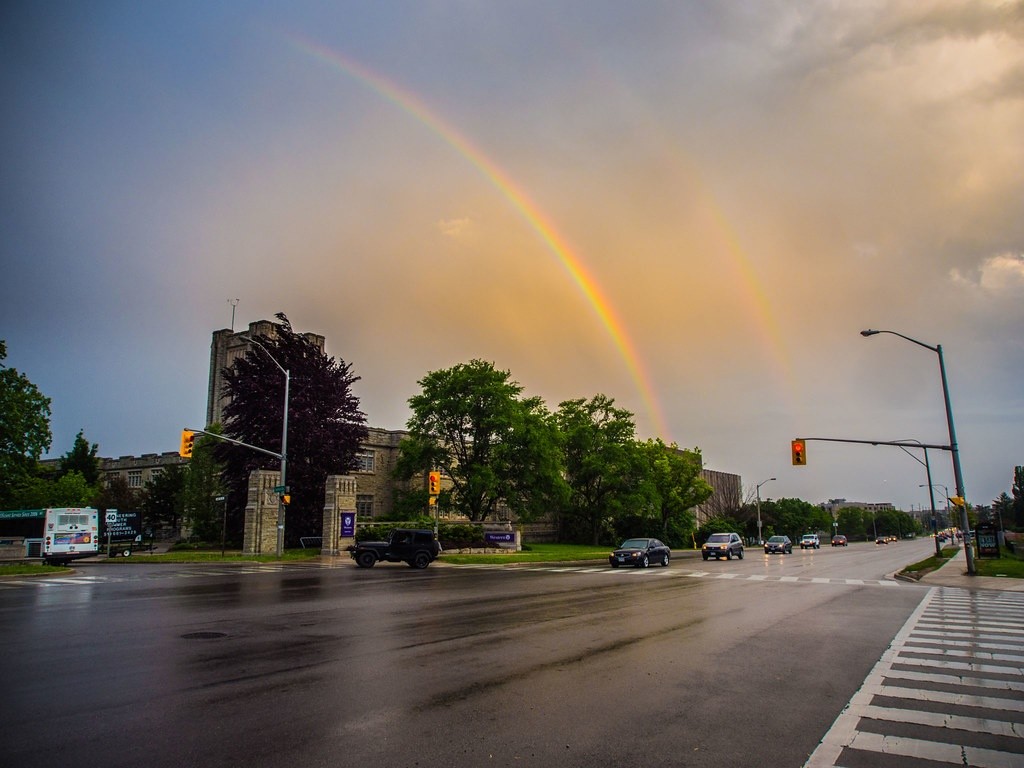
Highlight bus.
[0,505,99,565]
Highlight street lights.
[861,329,977,573]
[919,484,954,545]
[757,478,776,546]
[872,439,941,558]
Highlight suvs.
[702,533,744,561]
[800,535,820,548]
[351,528,442,568]
[764,535,793,554]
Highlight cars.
[609,538,671,568]
[876,536,897,545]
[831,535,847,547]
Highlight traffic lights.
[790,439,807,465]
[428,471,441,495]
[280,495,291,503]
[430,498,438,505]
[240,335,290,556]
[950,497,964,505]
[180,431,194,458]
